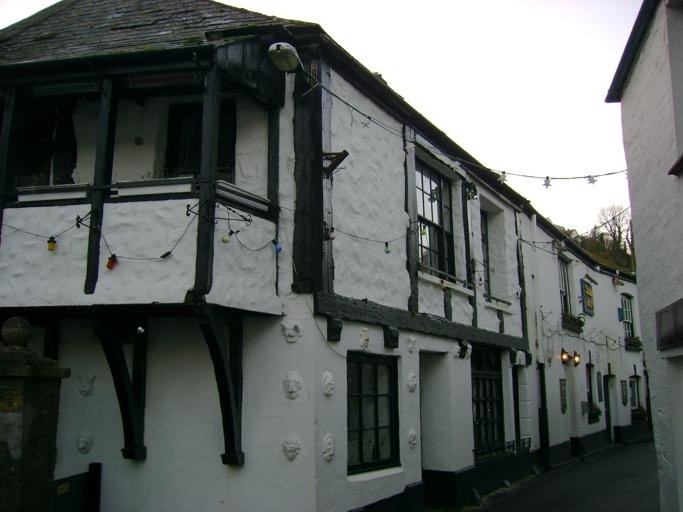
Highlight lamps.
[222,230,234,243]
[574,351,580,366]
[562,347,568,363]
[440,280,446,289]
[269,41,320,98]
[329,227,336,240]
[47,236,56,250]
[422,226,427,236]
[107,254,116,268]
[384,242,391,253]
[273,239,281,253]
